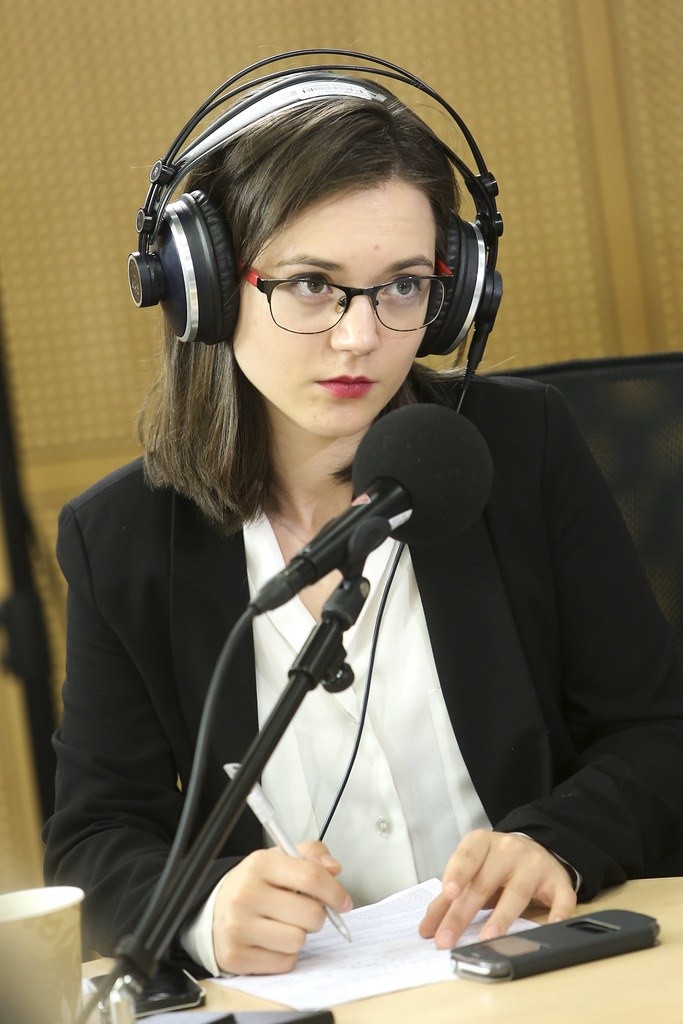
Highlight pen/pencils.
[223,762,354,943]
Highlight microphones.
[251,404,496,616]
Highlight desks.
[187,876,683,1024]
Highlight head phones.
[127,49,503,358]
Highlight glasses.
[237,259,455,332]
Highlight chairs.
[495,351,683,641]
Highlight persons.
[41,48,682,976]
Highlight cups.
[0,885,85,1024]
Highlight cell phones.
[450,909,661,982]
[88,968,206,1024]
